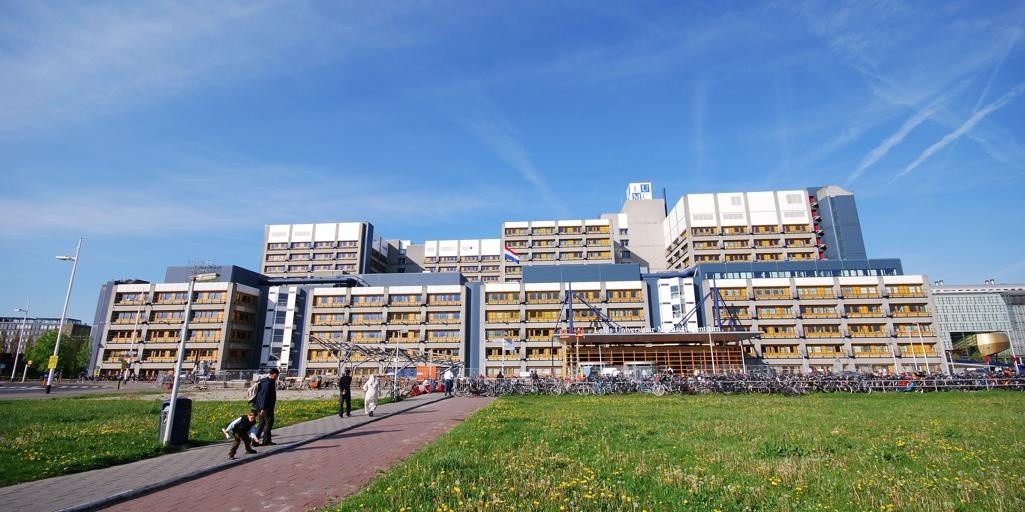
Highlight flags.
[504,244,520,265]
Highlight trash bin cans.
[158,397,192,445]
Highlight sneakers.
[228,441,277,460]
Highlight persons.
[530,370,534,379]
[362,373,379,416]
[534,370,539,378]
[497,371,504,381]
[255,368,279,445]
[338,369,353,418]
[220,408,258,460]
[444,369,454,396]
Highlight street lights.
[1000,329,1019,373]
[945,351,956,373]
[44,237,84,393]
[123,289,147,385]
[162,271,221,448]
[9,298,30,382]
[889,319,931,376]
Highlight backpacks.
[246,380,264,404]
